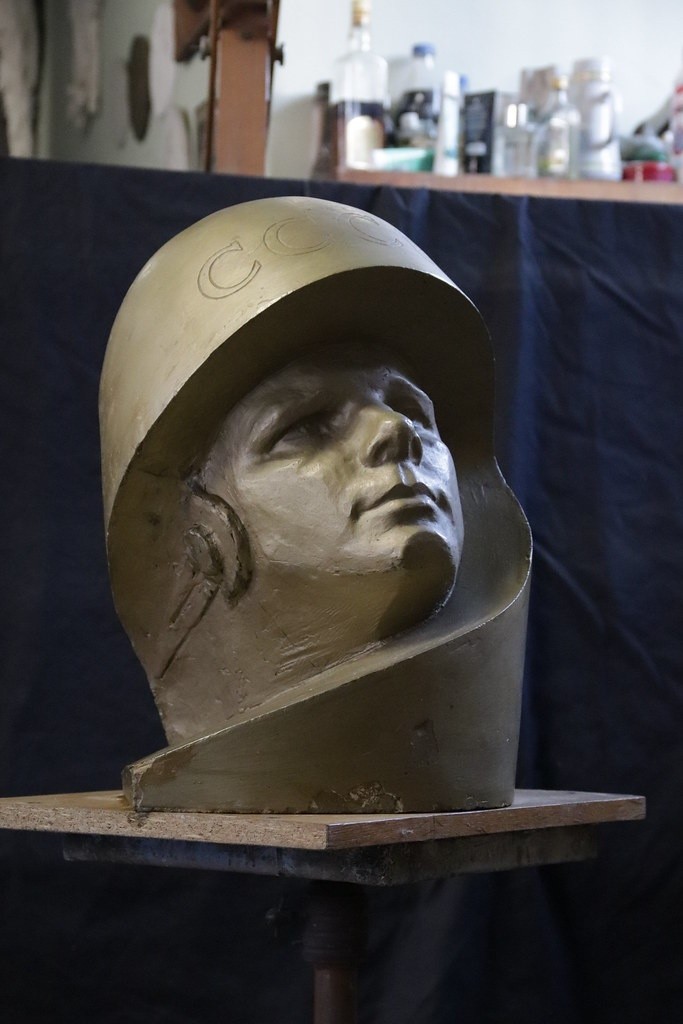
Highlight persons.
[182,343,463,638]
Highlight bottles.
[491,52,624,180]
[398,42,440,130]
[328,0,390,174]
